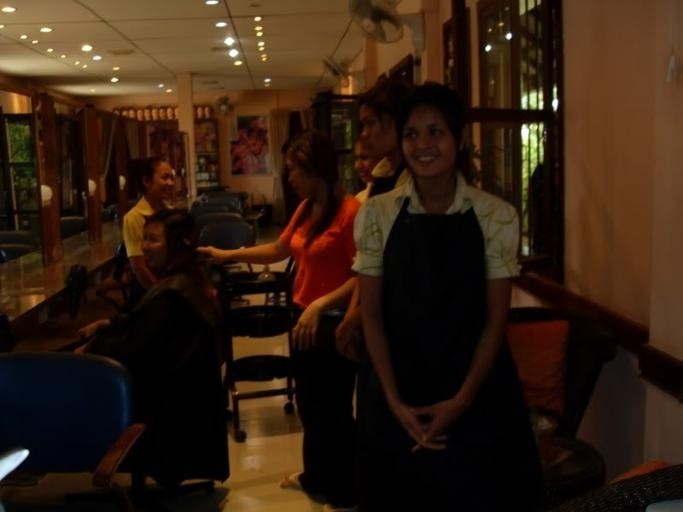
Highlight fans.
[323,55,367,92]
[212,96,235,116]
[349,0,425,50]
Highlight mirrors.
[0,85,144,287]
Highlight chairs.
[503,305,615,439]
[0,352,145,512]
[558,463,683,512]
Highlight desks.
[530,433,606,503]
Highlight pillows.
[500,318,571,413]
[609,459,673,484]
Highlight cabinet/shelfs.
[144,118,220,194]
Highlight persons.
[197,128,362,512]
[72,206,230,482]
[121,155,180,286]
[334,78,418,512]
[354,82,540,510]
[343,134,378,205]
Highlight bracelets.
[238,245,247,259]
